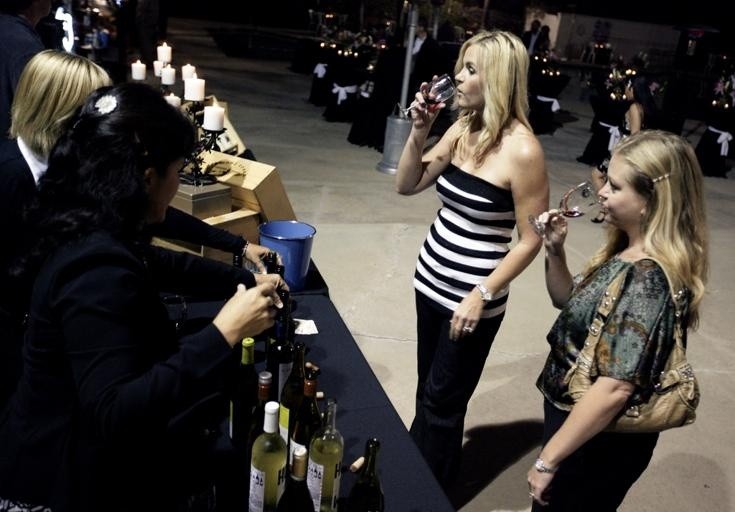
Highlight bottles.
[287,365,322,475]
[346,439,384,511]
[248,401,288,512]
[276,265,284,279]
[266,251,277,274]
[274,446,314,512]
[279,341,305,446]
[229,337,258,450]
[305,397,345,512]
[244,372,273,471]
[266,289,294,404]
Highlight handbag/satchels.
[567,256,700,429]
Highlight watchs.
[536,455,560,473]
[476,280,493,302]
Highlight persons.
[522,19,541,58]
[1,50,282,274]
[591,74,657,224]
[0,0,53,133]
[529,131,708,512]
[1,83,289,512]
[410,14,440,80]
[535,25,550,59]
[394,30,549,498]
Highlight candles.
[131,57,147,81]
[157,41,172,66]
[181,63,196,82]
[183,73,206,103]
[202,102,226,132]
[160,64,177,86]
[153,60,164,78]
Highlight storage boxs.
[182,148,298,225]
[150,205,262,270]
[170,182,234,221]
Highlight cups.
[527,180,598,246]
[397,74,456,122]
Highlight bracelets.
[242,241,249,256]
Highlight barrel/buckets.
[256,220,318,290]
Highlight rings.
[529,492,534,500]
[463,326,472,333]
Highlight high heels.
[592,211,605,223]
